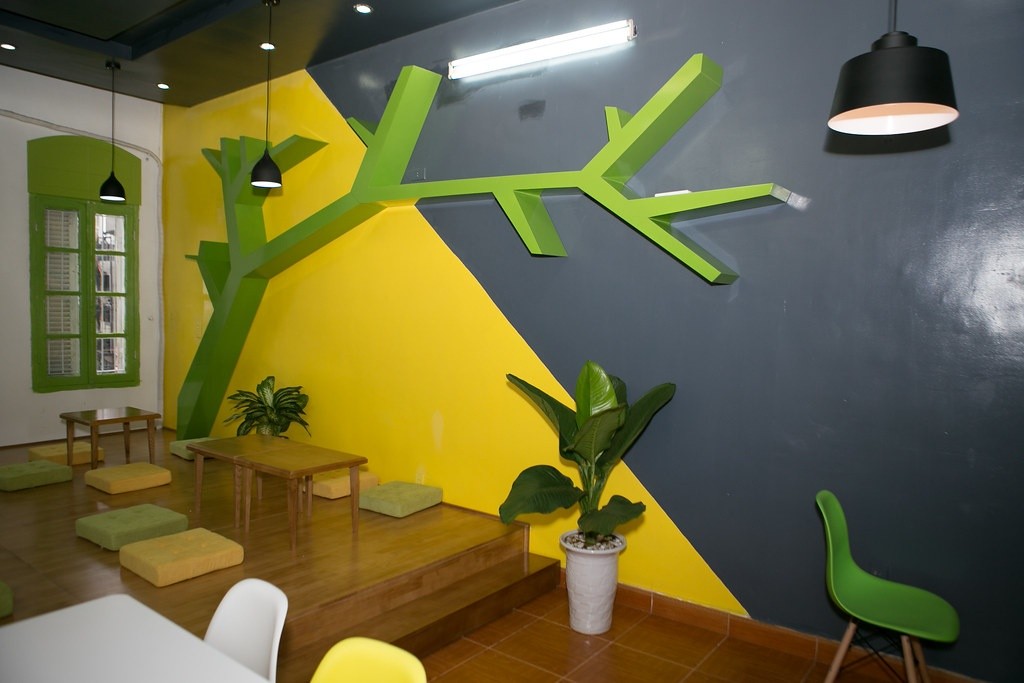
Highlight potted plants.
[221,376,313,441]
[499,360,677,635]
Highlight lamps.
[829,0,962,137]
[447,20,637,79]
[251,0,281,188]
[99,61,126,202]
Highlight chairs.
[311,635,429,683]
[203,576,290,681]
[814,489,959,683]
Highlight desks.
[0,593,272,682]
[60,406,163,470]
[188,435,370,550]
